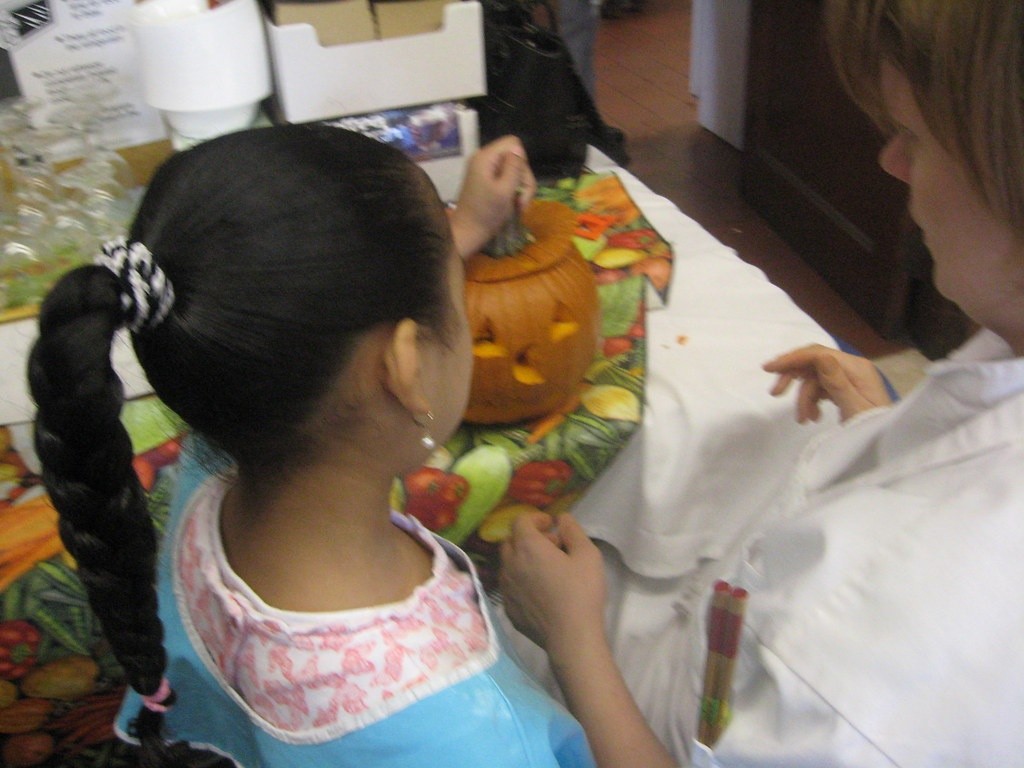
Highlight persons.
[27,125,600,768]
[469,1,632,177]
[500,0,1024,767]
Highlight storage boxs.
[259,0,487,125]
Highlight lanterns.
[462,216,599,423]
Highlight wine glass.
[0,92,136,304]
